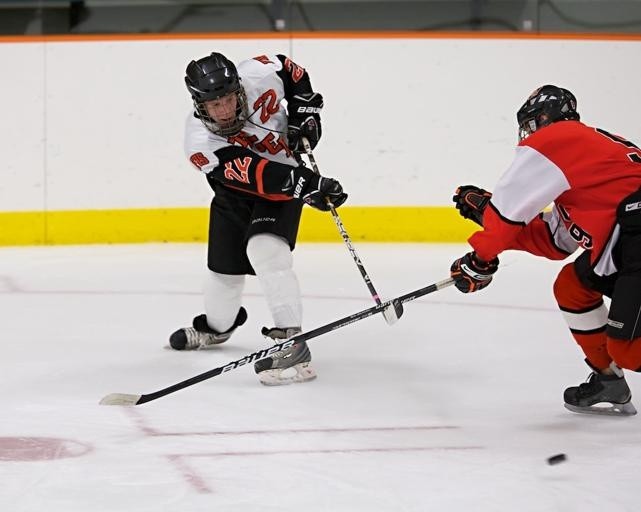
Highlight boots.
[563,357,632,407]
[170,306,248,350]
[254,326,311,374]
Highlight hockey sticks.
[301,137,403,326]
[98,277,456,407]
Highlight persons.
[170,51,346,372]
[450,82,641,408]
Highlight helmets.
[517,85,580,129]
[185,52,240,103]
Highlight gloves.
[282,168,348,212]
[451,251,499,293]
[287,93,324,153]
[453,185,492,226]
